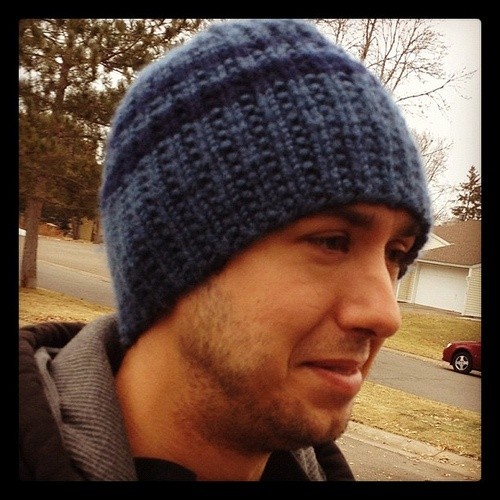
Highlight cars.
[441,339,481,373]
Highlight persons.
[17,16,435,481]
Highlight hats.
[99,20,429,346]
